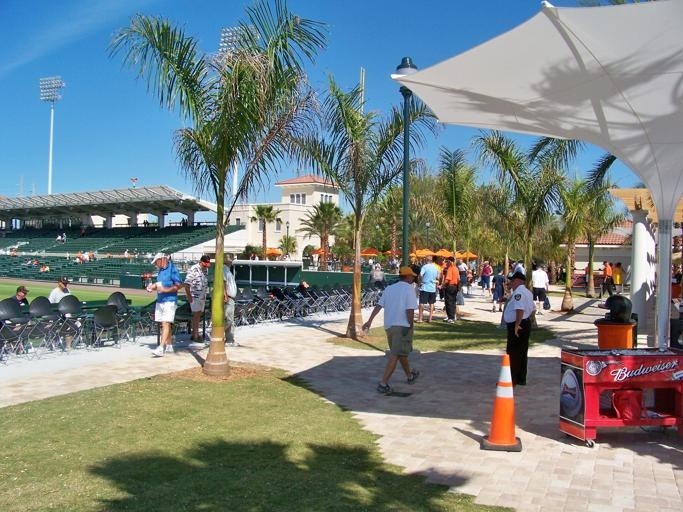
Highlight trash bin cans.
[361,266,370,273]
[593,295,638,350]
[341,265,350,272]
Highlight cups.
[155,281,162,294]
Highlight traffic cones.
[480,352,522,452]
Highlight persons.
[3,285,35,353]
[49,277,83,350]
[184,256,211,341]
[150,252,183,356]
[10,219,188,291]
[224,256,239,346]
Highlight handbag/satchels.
[543,297,549,310]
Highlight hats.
[16,286,29,293]
[58,276,69,284]
[507,271,524,280]
[151,252,167,265]
[200,256,210,267]
[224,253,235,263]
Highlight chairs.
[234,283,352,326]
[362,287,385,307]
[1,291,212,359]
[0,224,244,287]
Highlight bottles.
[145,278,152,292]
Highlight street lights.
[375,226,379,251]
[394,52,419,273]
[424,222,432,248]
[214,21,263,210]
[285,219,291,256]
[129,175,138,188]
[36,74,68,196]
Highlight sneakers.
[224,341,240,347]
[376,382,394,393]
[164,347,173,353]
[151,348,162,357]
[398,267,417,277]
[408,368,421,384]
